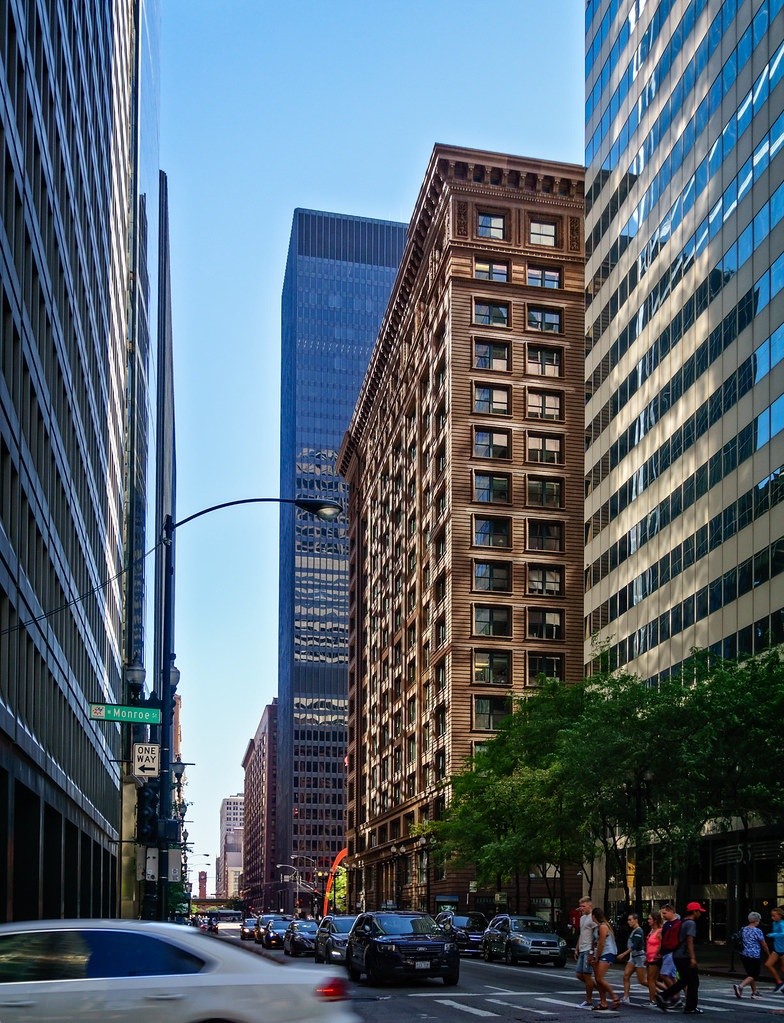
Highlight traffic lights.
[314,890,318,897]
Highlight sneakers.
[751,991,763,999]
[734,984,743,999]
[576,1000,593,1008]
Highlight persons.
[765,908,784,994]
[733,912,772,999]
[574,896,621,1010]
[617,902,706,1013]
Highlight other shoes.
[774,982,784,992]
[655,994,667,1011]
[642,1000,657,1006]
[668,999,684,1009]
[621,997,629,1003]
[683,1006,704,1013]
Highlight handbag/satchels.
[731,927,746,952]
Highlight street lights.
[290,854,318,919]
[390,843,406,909]
[275,864,298,916]
[126,653,186,922]
[344,862,356,916]
[624,768,655,920]
[157,494,344,922]
[419,833,436,914]
[318,868,340,919]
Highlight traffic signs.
[133,742,161,778]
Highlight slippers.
[611,997,620,1009]
[593,1004,608,1010]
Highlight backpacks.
[662,918,693,952]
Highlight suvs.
[345,910,460,988]
[314,912,360,964]
[254,914,286,944]
[482,914,567,969]
[434,910,489,955]
[199,919,219,934]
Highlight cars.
[239,918,257,939]
[0,918,364,1023]
[263,919,292,949]
[282,921,321,957]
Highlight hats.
[687,902,706,912]
